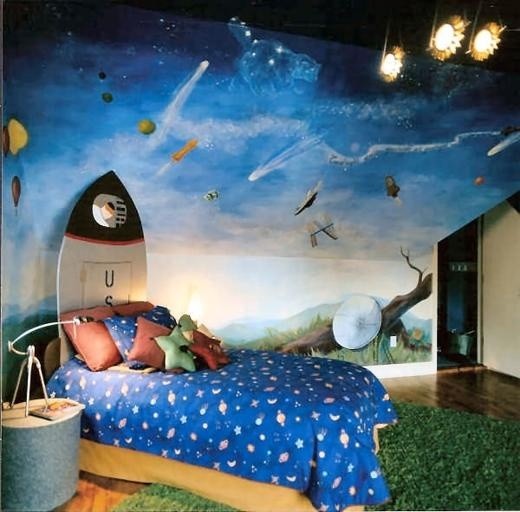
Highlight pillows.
[60,301,231,373]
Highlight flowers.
[407,325,430,349]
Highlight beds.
[43,335,398,508]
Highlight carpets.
[108,399,520,511]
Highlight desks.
[2,397,84,512]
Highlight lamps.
[466,5,507,63]
[425,7,472,61]
[378,11,405,84]
[8,314,87,415]
